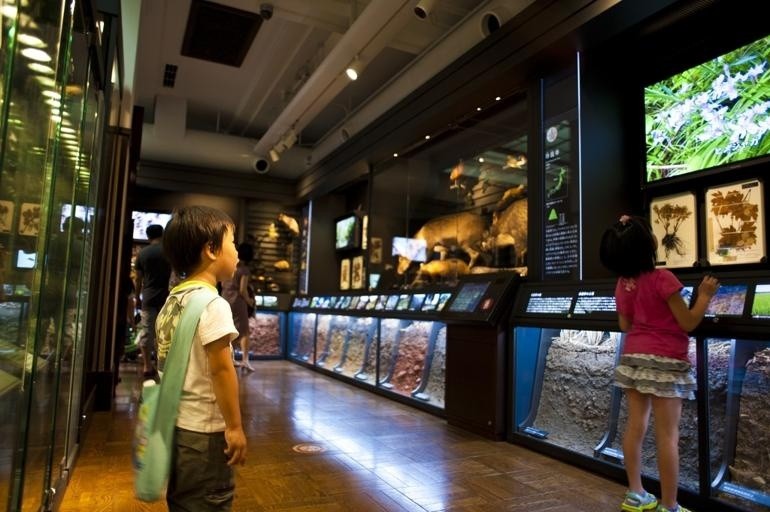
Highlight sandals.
[143,368,155,379]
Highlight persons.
[134,224,173,380]
[221,243,256,373]
[600,216,721,512]
[35,216,82,361]
[154,204,247,512]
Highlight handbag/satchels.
[131,385,182,501]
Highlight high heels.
[234,360,255,372]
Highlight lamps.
[247,154,270,175]
[413,0,435,20]
[345,55,368,81]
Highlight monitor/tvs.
[13,248,47,271]
[448,280,490,312]
[131,208,173,245]
[263,295,279,308]
[516,275,770,327]
[644,34,770,182]
[255,295,263,308]
[58,200,96,235]
[289,289,454,315]
[334,212,363,254]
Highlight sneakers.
[620,490,691,512]
